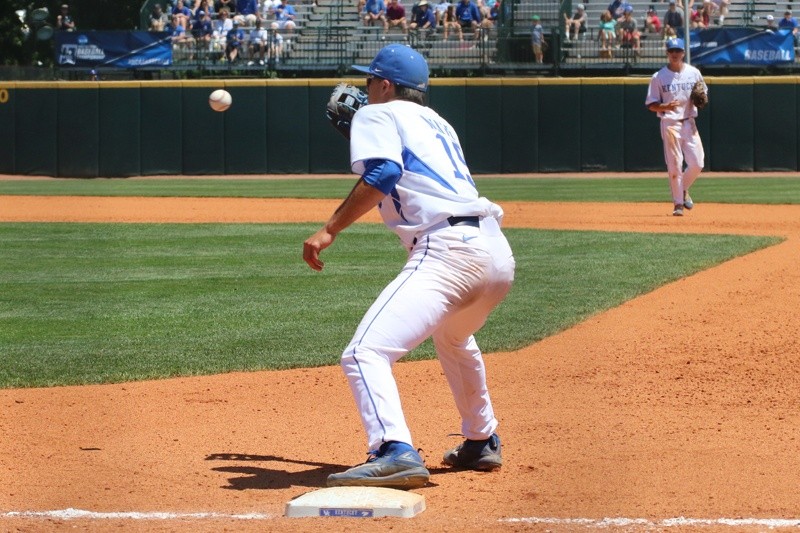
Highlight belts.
[413,215,479,245]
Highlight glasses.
[667,48,683,53]
[366,75,375,87]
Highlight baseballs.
[208,88,233,112]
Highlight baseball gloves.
[691,80,709,109]
[326,81,369,141]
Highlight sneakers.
[684,190,693,209]
[326,441,429,489]
[673,204,684,216]
[443,433,502,471]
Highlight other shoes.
[175,48,285,69]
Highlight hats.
[352,44,428,92]
[146,0,795,41]
[666,38,684,51]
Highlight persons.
[302,44,516,486]
[148,0,297,65]
[763,10,798,54]
[645,38,708,216]
[558,0,730,57]
[57,4,77,33]
[88,70,99,81]
[530,15,544,64]
[358,0,500,47]
